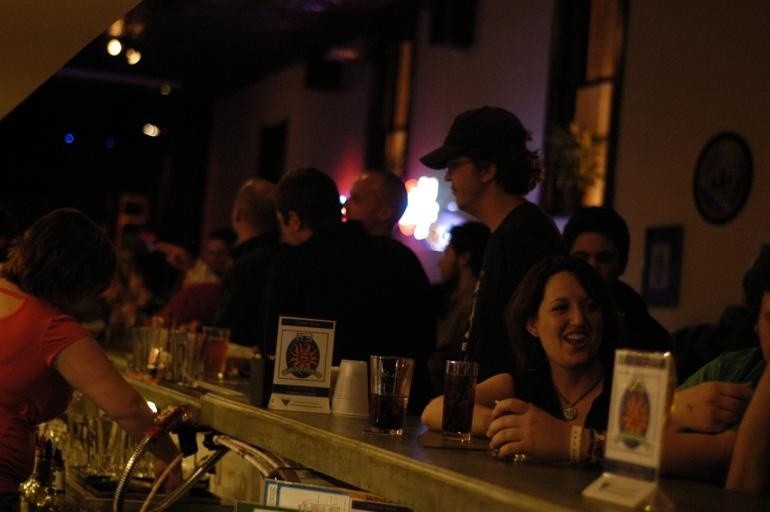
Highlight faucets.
[212,435,293,478]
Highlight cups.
[441,359,479,443]
[369,354,414,436]
[126,320,231,391]
[64,396,154,480]
[331,359,368,418]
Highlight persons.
[1,105,770,512]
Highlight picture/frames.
[640,225,684,308]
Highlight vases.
[552,183,584,215]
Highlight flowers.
[550,119,604,185]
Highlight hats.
[420,105,528,171]
